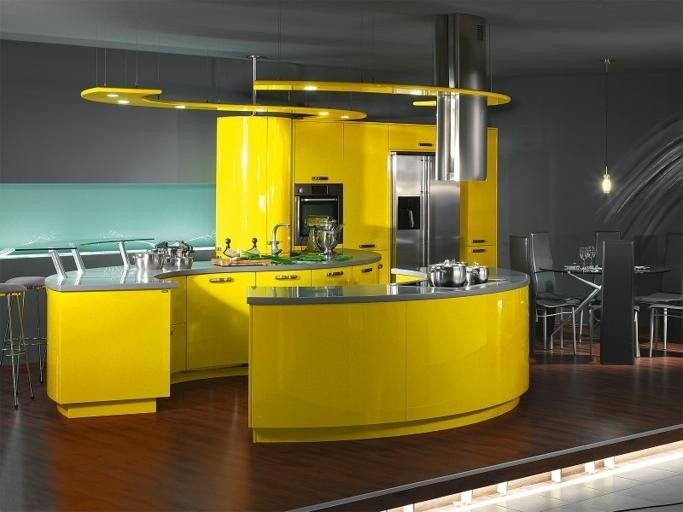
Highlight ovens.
[293,184,343,246]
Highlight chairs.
[504,222,681,366]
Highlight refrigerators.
[391,153,460,284]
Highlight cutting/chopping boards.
[210,258,271,266]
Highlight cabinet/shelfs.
[185,271,255,374]
[350,261,382,285]
[159,274,187,376]
[255,267,311,288]
[311,265,350,287]
[389,123,436,152]
[458,246,497,275]
[291,120,343,184]
[342,121,389,252]
[460,127,498,246]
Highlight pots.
[425,258,489,288]
[134,271,162,282]
[130,240,193,270]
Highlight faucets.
[271,223,291,257]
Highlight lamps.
[599,57,612,194]
[79,0,367,123]
[251,0,511,110]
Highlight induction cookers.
[392,274,505,289]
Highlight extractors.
[431,15,490,184]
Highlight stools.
[0,282,34,409]
[5,275,46,383]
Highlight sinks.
[288,260,330,265]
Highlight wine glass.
[317,226,341,257]
[578,245,595,269]
